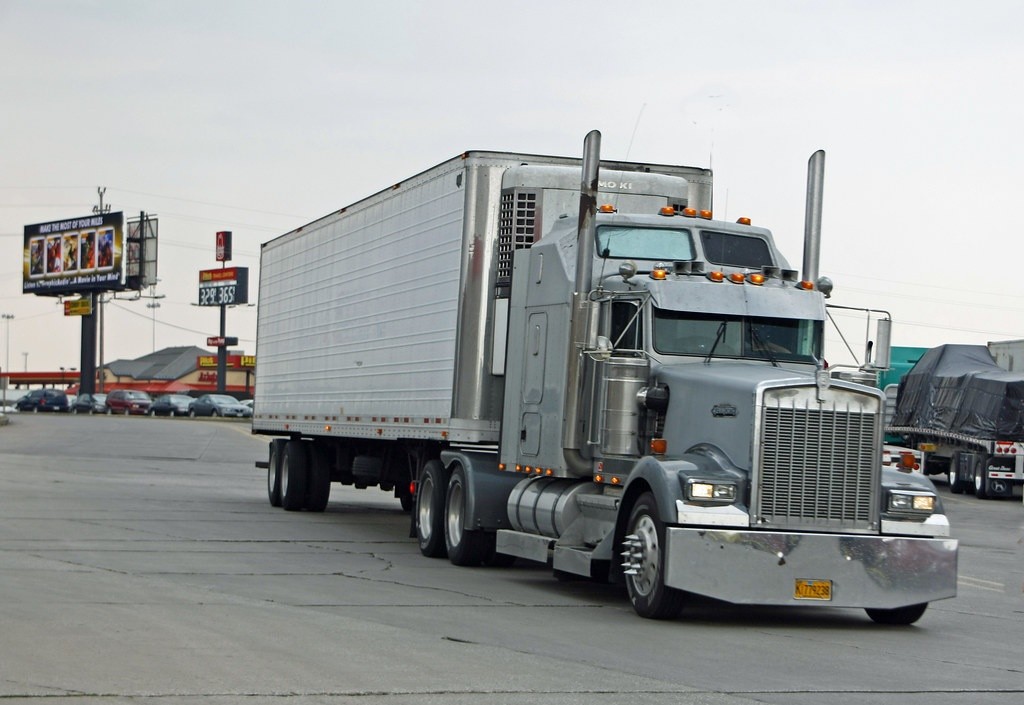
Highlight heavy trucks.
[875,344,1024,501]
[248,131,962,629]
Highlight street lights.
[2,313,15,373]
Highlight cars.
[71,393,109,416]
[105,390,153,416]
[187,394,251,418]
[15,388,69,414]
[148,394,197,417]
